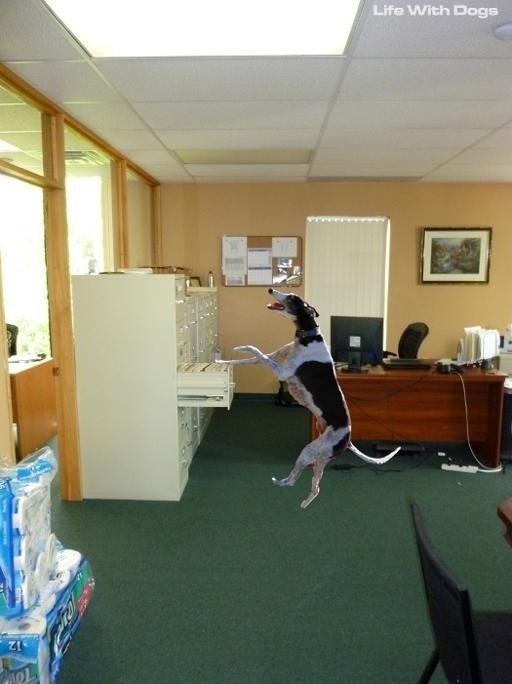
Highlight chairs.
[6,323,17,356]
[383,322,428,358]
[410,501,512,683]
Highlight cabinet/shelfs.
[71,274,237,501]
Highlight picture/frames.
[419,225,492,284]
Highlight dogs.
[213,288,402,509]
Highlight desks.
[496,498,512,546]
[310,358,509,468]
[9,355,57,460]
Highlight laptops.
[382,358,430,370]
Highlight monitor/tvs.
[331,315,383,372]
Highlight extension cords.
[441,463,478,473]
